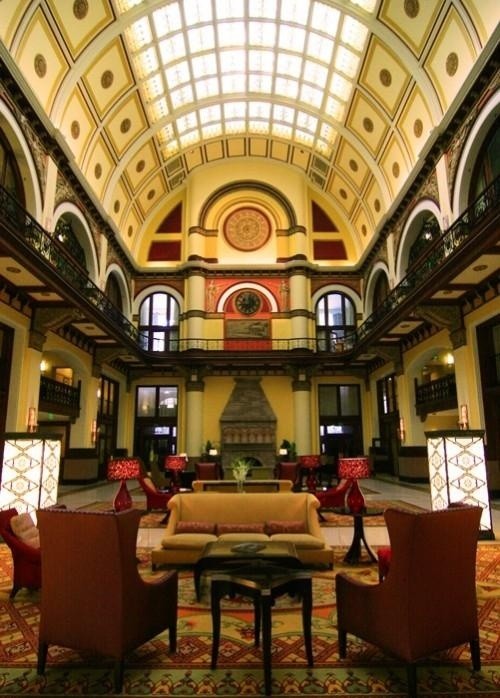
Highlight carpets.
[125,486,383,495]
[75,500,431,528]
[1,542,499,697]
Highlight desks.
[209,569,315,698]
[192,479,293,493]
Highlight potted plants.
[232,460,249,492]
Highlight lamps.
[301,455,320,491]
[164,456,186,493]
[92,420,97,442]
[28,407,38,433]
[39,223,70,254]
[338,457,379,565]
[107,458,142,512]
[457,403,468,429]
[423,429,496,541]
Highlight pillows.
[175,520,305,535]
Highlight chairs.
[0,505,178,695]
[335,499,484,697]
[195,462,217,480]
[139,474,174,511]
[275,462,300,486]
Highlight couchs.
[151,492,334,572]
